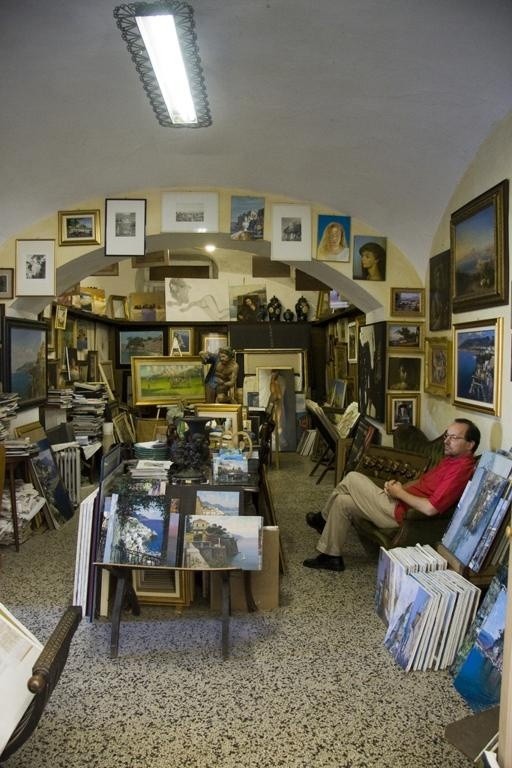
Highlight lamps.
[113,0,212,128]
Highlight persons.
[191,433,208,461]
[237,297,257,322]
[199,346,239,404]
[304,418,480,572]
[317,221,349,262]
[168,280,229,319]
[390,359,417,390]
[359,242,385,281]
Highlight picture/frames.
[103,198,146,257]
[58,210,100,245]
[115,326,168,368]
[356,321,388,420]
[335,342,347,380]
[346,323,358,363]
[424,337,452,400]
[98,360,114,388]
[121,371,137,413]
[247,391,258,420]
[257,366,297,452]
[111,411,134,444]
[108,295,128,320]
[200,330,227,356]
[168,327,195,356]
[130,356,215,406]
[385,392,421,434]
[315,291,332,319]
[195,404,242,437]
[193,485,246,517]
[79,361,91,383]
[0,268,13,299]
[70,286,106,315]
[88,351,98,382]
[3,316,48,411]
[391,287,426,318]
[133,250,171,268]
[450,317,503,418]
[57,318,78,361]
[55,304,68,331]
[387,321,426,355]
[449,178,510,314]
[14,239,57,297]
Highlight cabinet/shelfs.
[92,561,260,663]
[0,456,25,553]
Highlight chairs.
[344,424,468,551]
[444,705,501,767]
[0,600,83,762]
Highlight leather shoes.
[306,511,325,534]
[303,554,345,572]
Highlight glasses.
[444,434,463,441]
